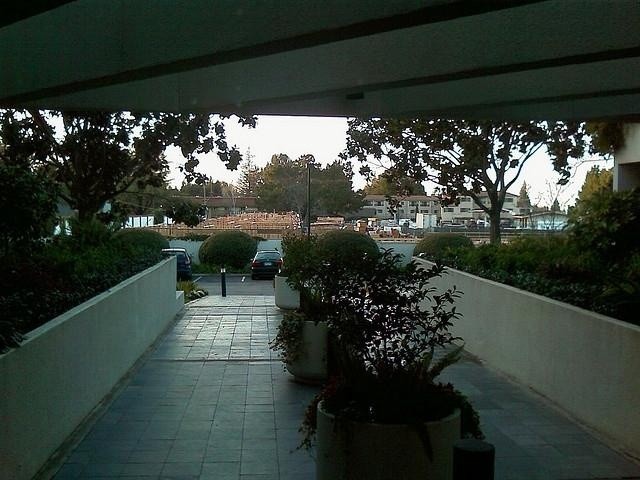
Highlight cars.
[161,248,191,278]
[250,250,285,280]
[377,218,510,228]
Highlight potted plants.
[288,248,483,480]
[273,229,318,310]
[269,227,370,387]
